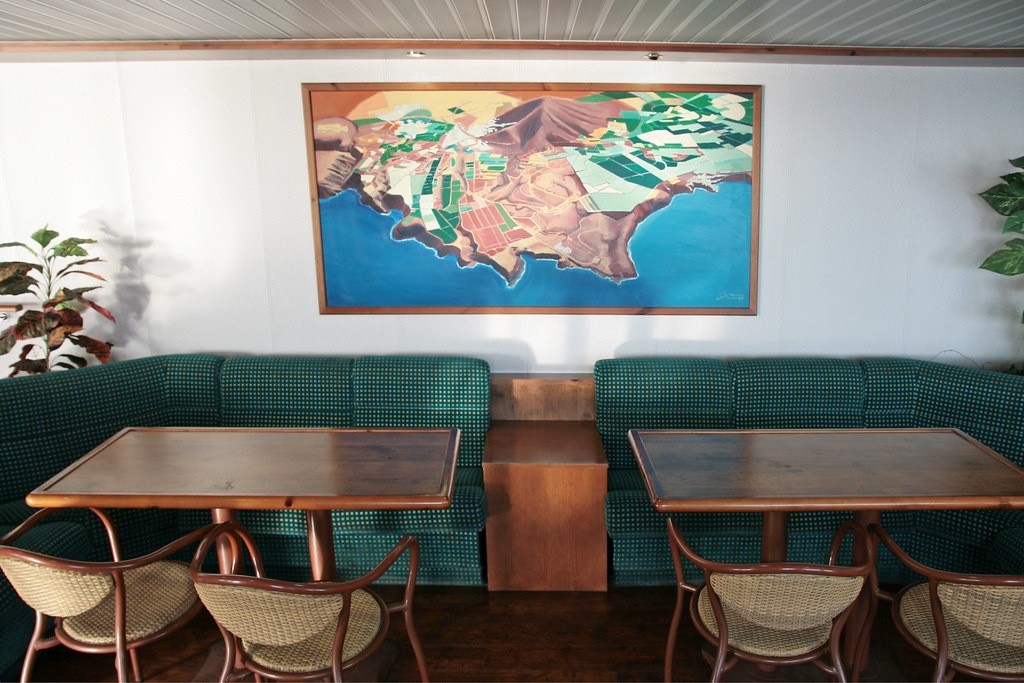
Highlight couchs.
[594,357,1024,587]
[1,353,494,676]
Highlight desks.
[628,427,1024,683]
[24,427,460,683]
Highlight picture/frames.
[301,81,763,316]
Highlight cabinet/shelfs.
[483,418,610,594]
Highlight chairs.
[664,515,875,683]
[0,507,242,683]
[848,515,1024,683]
[190,520,429,683]
